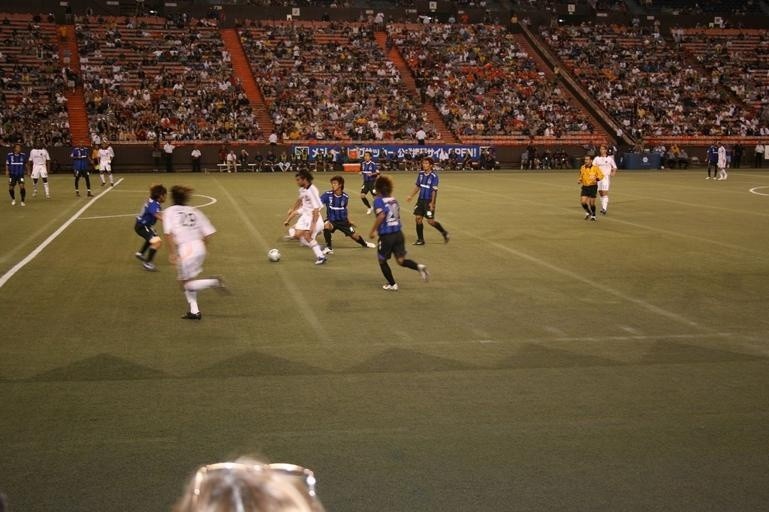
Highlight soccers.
[268,249,280,261]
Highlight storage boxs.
[342,163,361,172]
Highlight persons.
[68,139,95,198]
[592,144,618,214]
[170,457,332,512]
[319,176,376,254]
[286,171,304,217]
[27,141,51,201]
[97,135,118,188]
[370,178,431,291]
[576,156,604,222]
[295,170,325,265]
[160,185,232,323]
[357,151,382,215]
[134,184,166,271]
[405,157,451,247]
[2,1,768,180]
[3,144,30,206]
[283,210,325,240]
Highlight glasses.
[191,462,317,503]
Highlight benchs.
[0,14,766,143]
[216,163,328,173]
[399,159,501,172]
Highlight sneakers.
[411,240,424,245]
[443,232,451,243]
[181,312,201,320]
[367,206,374,215]
[135,252,146,260]
[366,241,377,248]
[314,246,335,265]
[383,283,399,291]
[20,202,26,206]
[584,209,606,223]
[417,264,431,282]
[12,199,16,205]
